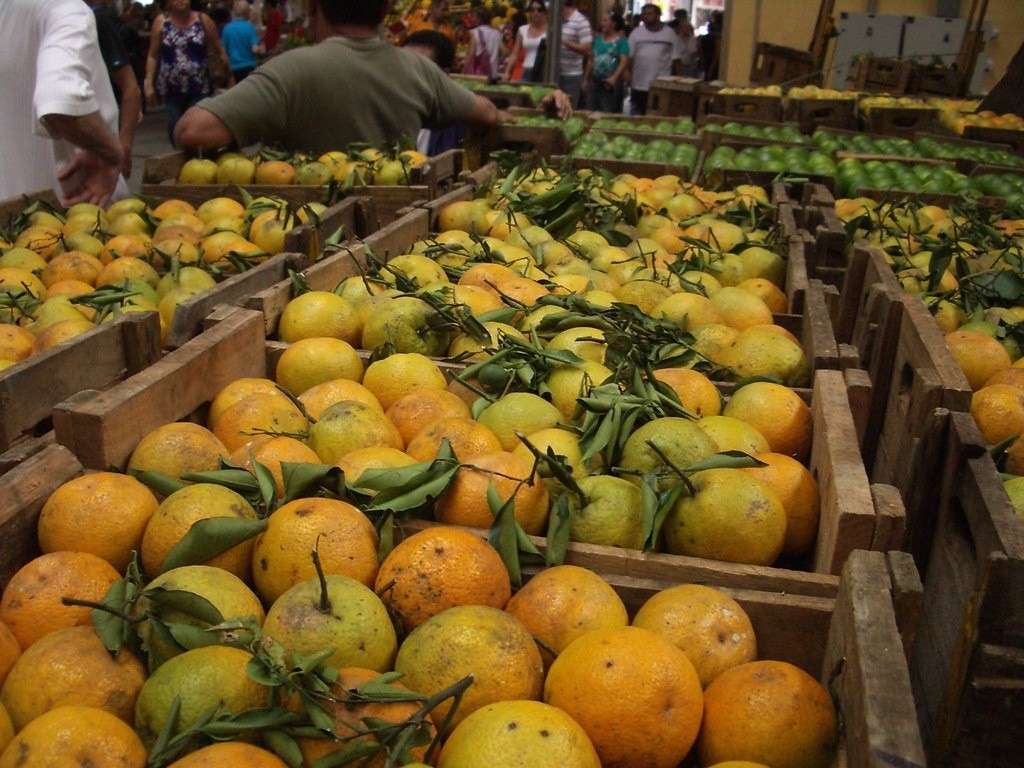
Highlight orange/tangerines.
[0,196,329,375]
[178,141,428,187]
[0,472,842,768]
[125,164,821,574]
[836,197,1024,522]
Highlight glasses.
[528,6,544,13]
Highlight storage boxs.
[0,51,1024,768]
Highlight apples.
[719,85,1024,138]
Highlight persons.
[0,0,129,210]
[508,12,527,82]
[463,8,507,85]
[399,30,570,164]
[86,0,229,180]
[502,0,547,82]
[560,0,593,108]
[174,0,518,157]
[407,0,456,43]
[582,10,630,113]
[624,3,724,115]
[221,0,265,82]
[250,0,291,50]
[974,42,1024,114]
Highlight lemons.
[450,77,554,104]
[497,114,1024,209]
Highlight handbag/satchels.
[465,27,492,81]
